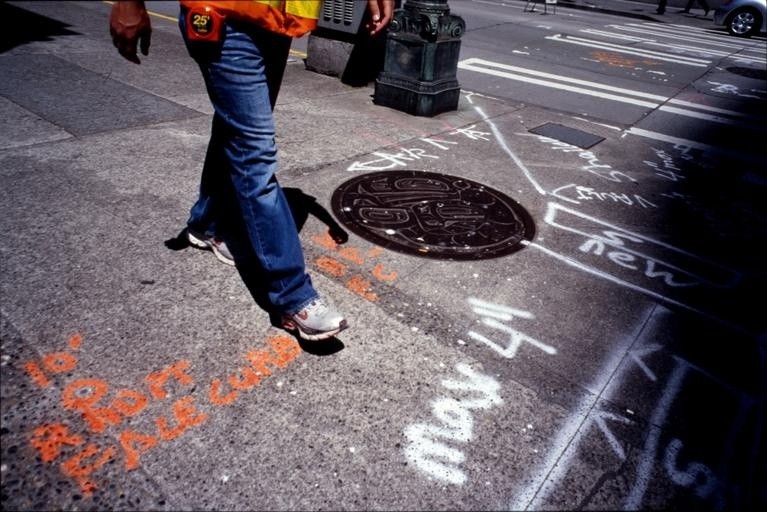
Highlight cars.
[711,0,766,38]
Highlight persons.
[110,0,395,341]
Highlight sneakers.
[276,296,348,341]
[187,226,238,266]
